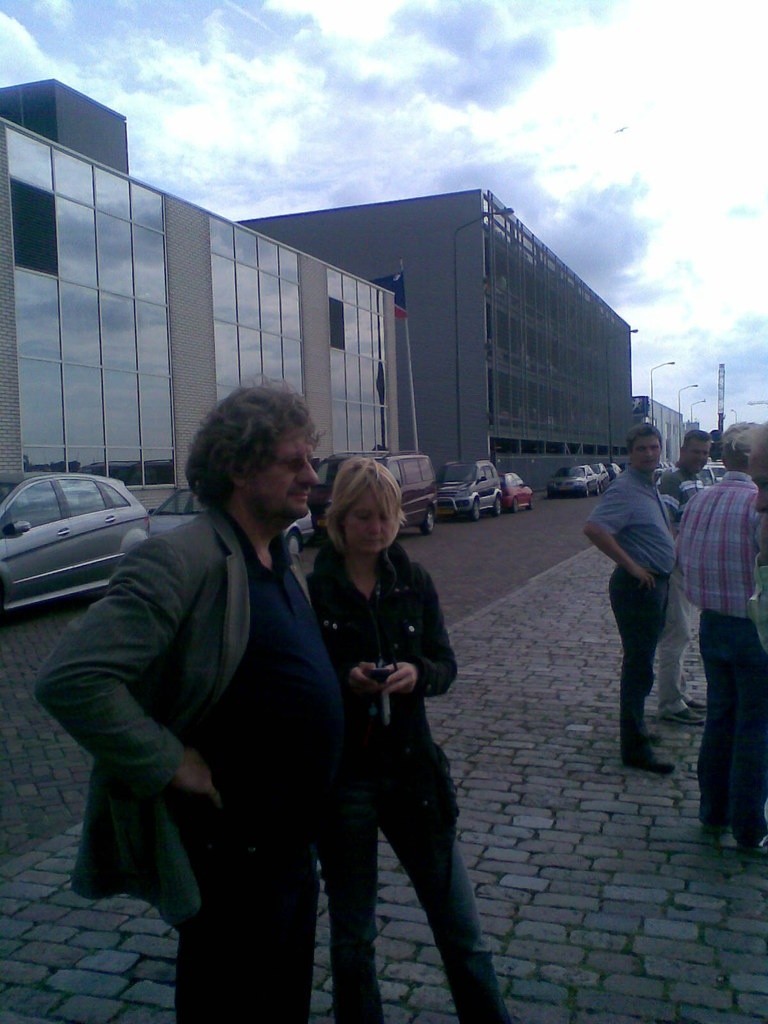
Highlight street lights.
[450,206,516,461]
[650,361,676,427]
[731,409,738,423]
[678,384,699,451]
[606,329,639,463]
[690,400,706,431]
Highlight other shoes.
[641,758,675,773]
[664,708,705,724]
[648,734,660,742]
[737,834,768,855]
[686,700,707,712]
[703,822,733,833]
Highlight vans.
[306,450,438,539]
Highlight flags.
[369,273,408,320]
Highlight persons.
[749,421,768,652]
[305,456,512,1024]
[34,385,343,1024]
[656,430,711,723]
[674,422,768,850]
[583,422,677,772]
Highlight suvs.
[0,471,162,616]
[435,458,503,521]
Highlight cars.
[545,462,628,500]
[654,459,727,488]
[147,486,316,556]
[498,471,536,514]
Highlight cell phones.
[368,668,390,683]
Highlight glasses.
[274,458,320,473]
